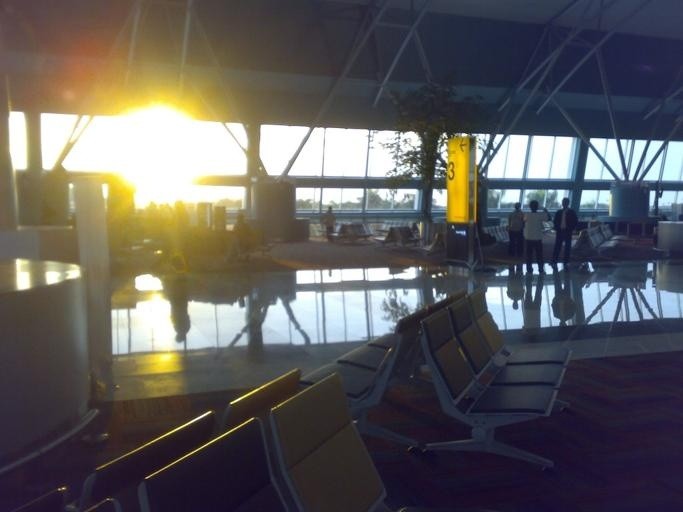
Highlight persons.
[507,264,525,310]
[172,299,190,343]
[552,197,579,265]
[322,204,337,245]
[551,265,577,327]
[523,275,543,328]
[522,198,550,274]
[507,201,524,259]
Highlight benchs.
[299,289,570,473]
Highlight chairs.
[482,216,683,268]
[15,366,387,508]
[328,224,446,257]
